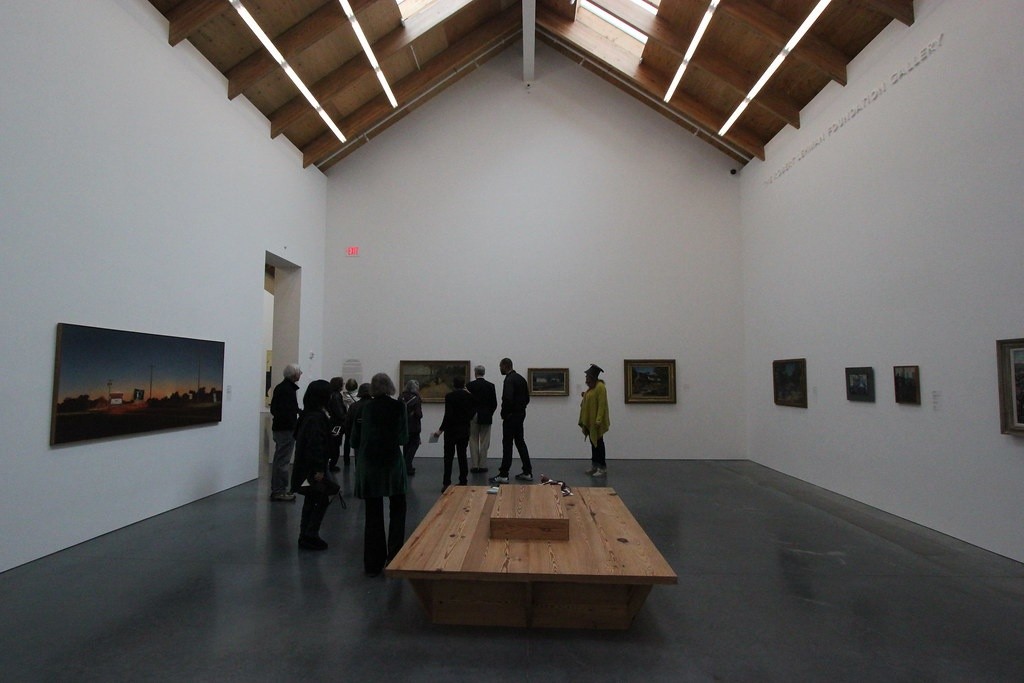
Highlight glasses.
[299,371,302,375]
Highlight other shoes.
[441,484,451,494]
[585,467,596,475]
[593,468,607,477]
[456,481,467,486]
[515,472,533,481]
[471,467,488,472]
[271,492,297,501]
[298,533,328,550]
[488,474,509,484]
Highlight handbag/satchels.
[312,455,346,510]
[349,402,357,448]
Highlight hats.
[585,364,604,380]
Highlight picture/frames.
[893,365,921,404]
[772,358,809,409]
[844,366,875,402]
[624,358,677,405]
[527,367,570,397]
[399,359,471,404]
[995,338,1024,437]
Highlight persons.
[269,365,304,502]
[349,372,409,578]
[466,365,498,475]
[433,376,477,494]
[326,377,374,470]
[397,379,423,476]
[290,380,335,549]
[578,364,611,478]
[488,358,534,484]
